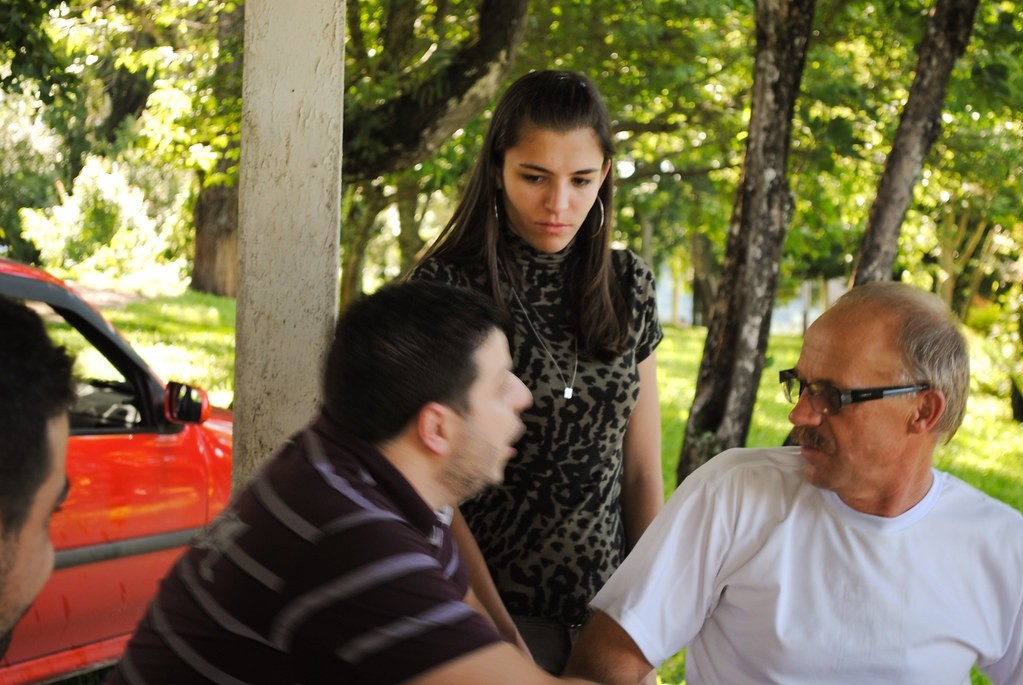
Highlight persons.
[109,287,599,685]
[402,70,662,675]
[1,294,77,661]
[568,283,1022,684]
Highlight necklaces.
[500,259,577,400]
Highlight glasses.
[779,368,931,415]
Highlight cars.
[0,253,238,685]
